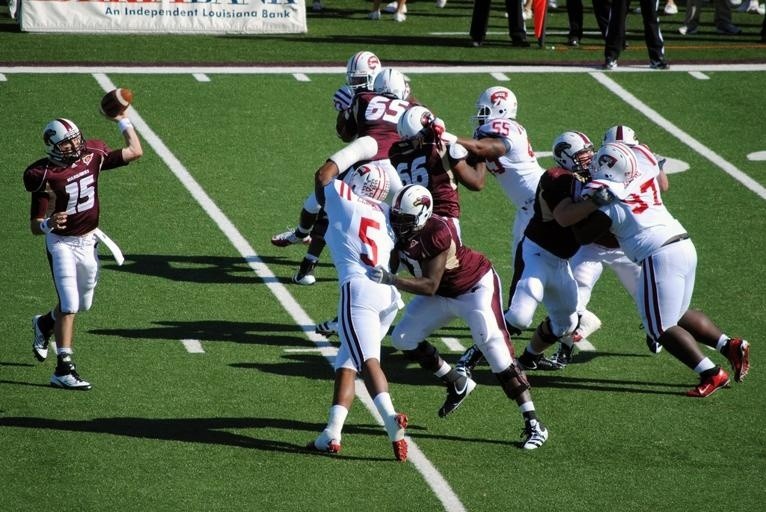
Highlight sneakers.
[391,413,409,462]
[315,320,339,338]
[271,231,313,247]
[727,338,751,382]
[31,315,50,362]
[688,365,731,396]
[307,429,341,454]
[520,417,549,451]
[717,23,742,36]
[650,56,669,70]
[647,330,665,353]
[606,56,618,70]
[523,7,532,21]
[678,24,699,35]
[51,362,91,391]
[439,375,476,418]
[456,346,483,379]
[518,352,563,371]
[549,345,570,368]
[291,269,316,286]
[392,13,406,21]
[367,11,380,20]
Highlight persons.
[23,105,144,391]
[432,86,548,268]
[366,0,408,22]
[565,0,629,49]
[557,143,749,398]
[549,126,668,368]
[290,69,420,285]
[307,136,407,462]
[603,0,669,70]
[369,183,549,450]
[457,130,616,371]
[270,52,420,247]
[679,0,742,35]
[470,0,532,48]
[316,106,486,338]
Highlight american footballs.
[100,89,133,118]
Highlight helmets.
[346,50,382,92]
[373,69,411,100]
[390,184,433,240]
[44,117,85,167]
[349,163,390,205]
[472,86,517,124]
[602,125,640,147]
[590,143,637,184]
[552,131,593,175]
[397,106,445,143]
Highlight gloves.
[366,265,396,284]
[591,188,615,207]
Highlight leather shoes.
[469,36,482,47]
[519,38,530,47]
[569,37,581,46]
